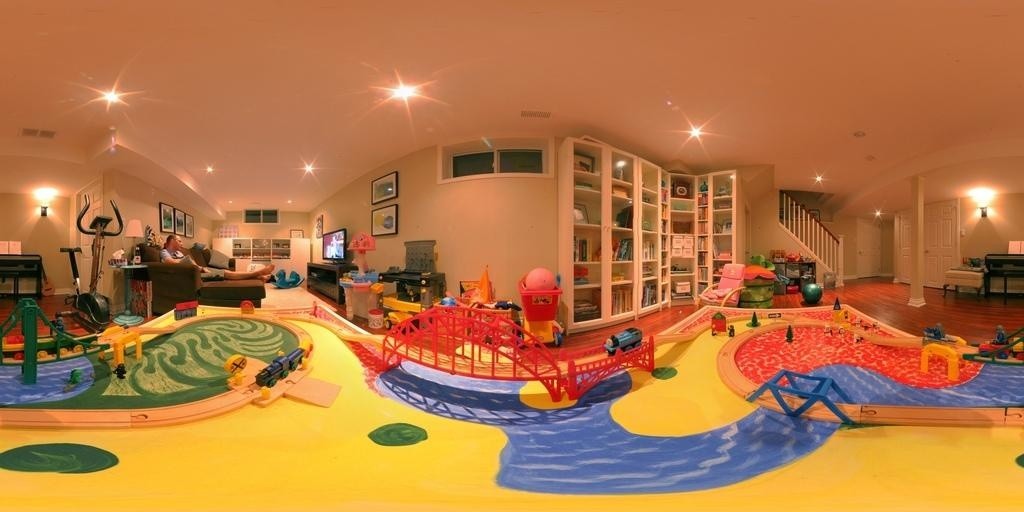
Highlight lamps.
[124,219,144,265]
[34,200,56,218]
[970,193,996,221]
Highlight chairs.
[696,264,746,308]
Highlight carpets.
[254,282,339,313]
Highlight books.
[1008,240,1024,254]
[595,238,633,261]
[0,241,22,255]
[661,179,668,302]
[697,175,710,297]
[643,240,654,260]
[574,301,601,322]
[593,287,633,315]
[642,285,656,307]
[574,234,594,263]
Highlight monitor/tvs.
[322,228,346,261]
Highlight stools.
[940,267,985,299]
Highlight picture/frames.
[160,202,173,232]
[184,213,194,239]
[372,170,398,204]
[369,205,399,237]
[290,229,305,239]
[172,208,185,236]
[809,209,821,222]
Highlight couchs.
[136,240,266,315]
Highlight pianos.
[984,254,1024,303]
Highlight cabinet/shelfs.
[695,171,711,297]
[671,172,695,306]
[211,238,312,282]
[659,168,671,310]
[773,259,817,296]
[711,168,752,299]
[306,262,358,305]
[638,156,661,319]
[561,137,640,325]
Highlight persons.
[160,235,276,281]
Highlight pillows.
[208,247,230,270]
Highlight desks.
[980,253,1023,306]
[113,262,149,326]
[382,269,444,313]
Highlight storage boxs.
[519,279,562,322]
[346,281,397,320]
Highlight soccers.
[526,268,555,291]
[802,284,823,303]
